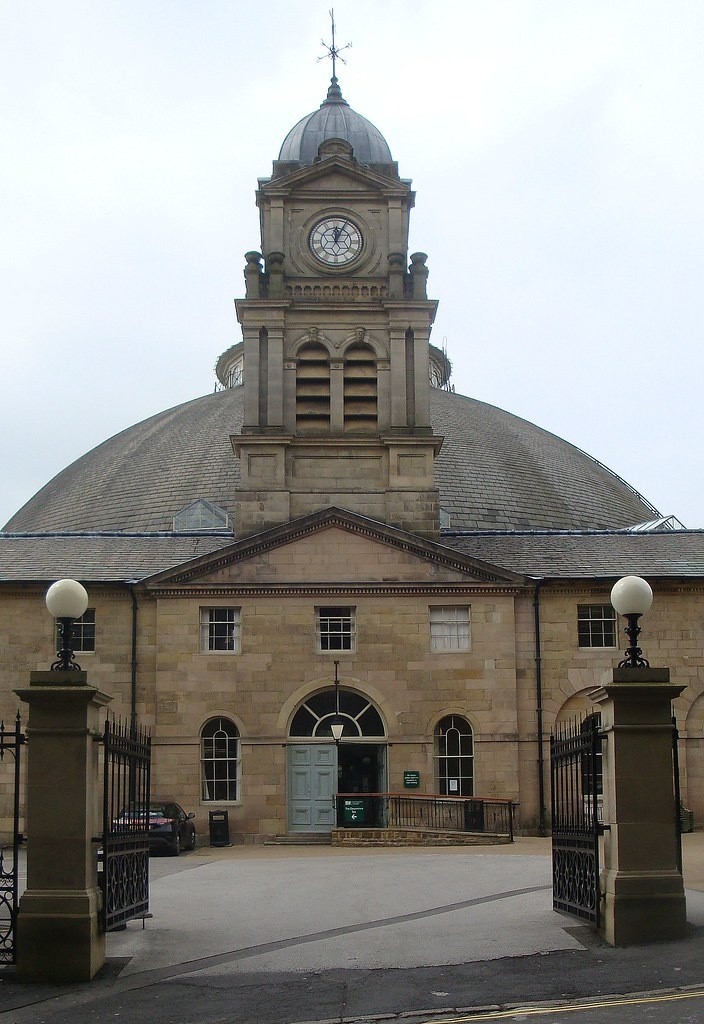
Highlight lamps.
[46,579,88,672]
[330,660,345,746]
[610,576,653,667]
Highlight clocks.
[310,217,363,266]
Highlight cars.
[112,801,196,857]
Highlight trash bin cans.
[464,799,485,832]
[208,809,230,847]
